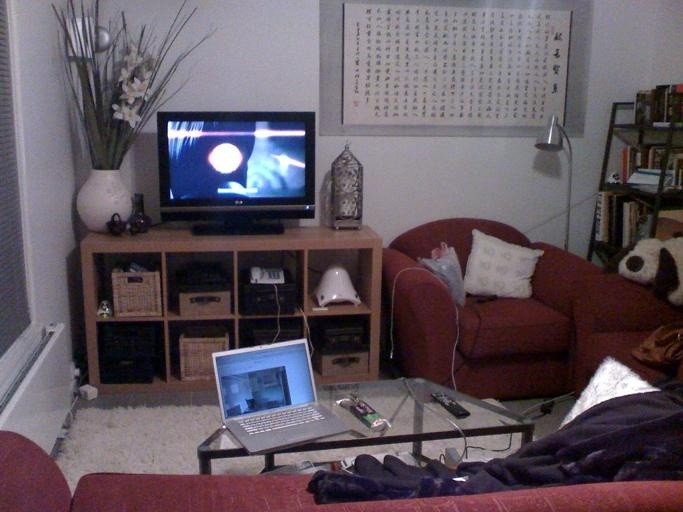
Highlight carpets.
[52,395,538,498]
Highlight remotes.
[430,390,470,418]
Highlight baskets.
[179,325,230,381]
[316,351,369,375]
[178,291,231,316]
[111,271,162,317]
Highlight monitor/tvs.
[156,110,316,236]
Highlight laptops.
[211,337,351,456]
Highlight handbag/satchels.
[631,324,683,366]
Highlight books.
[596,83,683,254]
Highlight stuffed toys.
[614,236,683,308]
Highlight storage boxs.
[240,269,296,315]
[313,342,370,378]
[175,283,232,317]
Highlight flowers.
[47,0,221,170]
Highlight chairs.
[380,215,604,401]
[571,268,682,399]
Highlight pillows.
[462,227,547,300]
[556,351,665,433]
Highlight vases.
[75,169,133,235]
[126,190,152,233]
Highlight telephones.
[249,267,285,284]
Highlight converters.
[540,398,554,414]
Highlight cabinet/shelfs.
[79,222,383,397]
[584,92,682,269]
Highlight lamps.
[532,114,575,252]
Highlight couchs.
[0,426,681,511]
